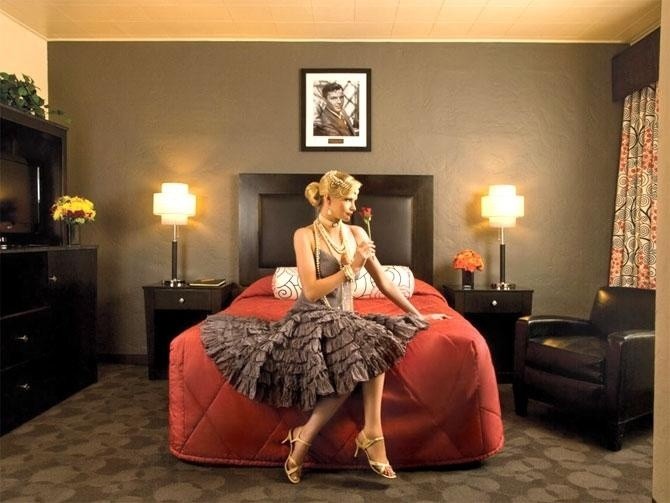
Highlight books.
[189,278,227,287]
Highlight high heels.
[282,426,312,484]
[353,430,397,479]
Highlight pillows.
[271,262,414,302]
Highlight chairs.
[513,285,656,450]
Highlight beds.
[166,173,507,467]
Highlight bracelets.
[342,264,356,281]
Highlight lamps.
[480,183,524,291]
[153,183,197,285]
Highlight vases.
[64,221,82,246]
[462,267,475,291]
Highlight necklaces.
[312,211,354,312]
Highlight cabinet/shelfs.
[43,244,99,399]
[1,251,47,433]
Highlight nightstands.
[141,282,234,383]
[441,282,533,378]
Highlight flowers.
[358,204,376,240]
[451,248,485,273]
[50,195,97,223]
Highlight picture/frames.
[298,68,372,153]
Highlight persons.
[314,82,357,136]
[200,169,454,483]
